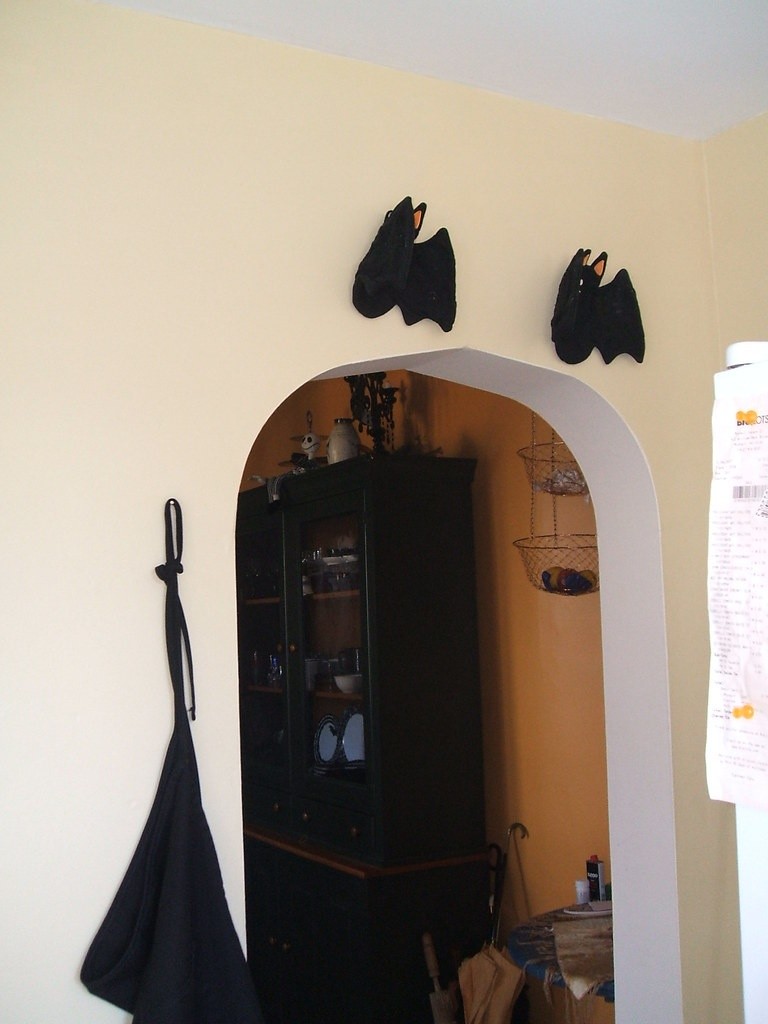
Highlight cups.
[244,546,362,693]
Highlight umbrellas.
[458,823,527,1024]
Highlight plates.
[343,554,360,563]
[323,556,344,566]
[311,704,365,766]
[564,900,612,917]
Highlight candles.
[386,380,392,389]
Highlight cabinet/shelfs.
[236,451,507,1024]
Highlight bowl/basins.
[333,675,361,693]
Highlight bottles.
[575,879,589,905]
[328,418,359,465]
[586,854,606,901]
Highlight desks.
[510,903,615,1024]
